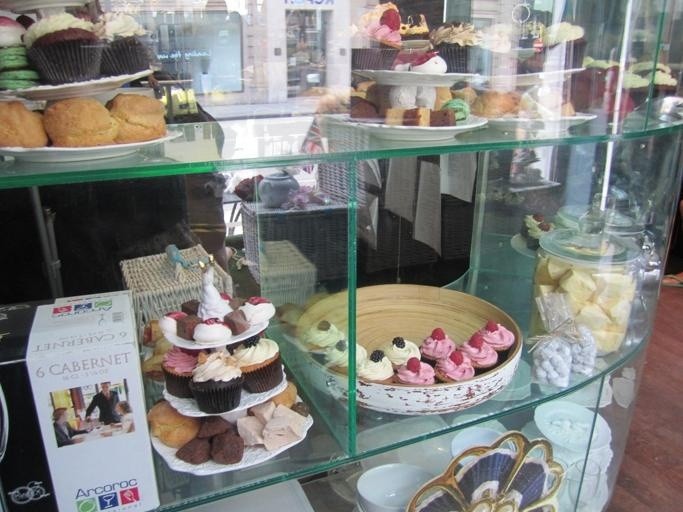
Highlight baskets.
[117,244,233,498]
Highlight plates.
[333,110,487,142]
[509,231,539,260]
[150,395,313,476]
[490,65,587,89]
[494,110,597,132]
[0,128,186,165]
[532,398,614,450]
[163,305,269,348]
[567,379,611,406]
[18,65,159,108]
[162,363,287,417]
[349,69,474,89]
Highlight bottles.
[527,193,644,355]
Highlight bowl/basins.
[449,426,517,470]
[293,280,525,418]
[355,466,438,509]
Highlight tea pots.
[259,171,296,209]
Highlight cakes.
[0,13,148,88]
[521,212,554,250]
[625,61,677,101]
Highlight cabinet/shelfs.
[1,0,681,511]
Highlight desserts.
[143,268,314,466]
[348,3,651,128]
[272,282,517,387]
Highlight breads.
[0,94,168,155]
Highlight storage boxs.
[24,288,163,512]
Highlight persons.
[52,408,91,446]
[51,69,229,284]
[85,382,120,425]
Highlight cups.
[544,457,601,511]
